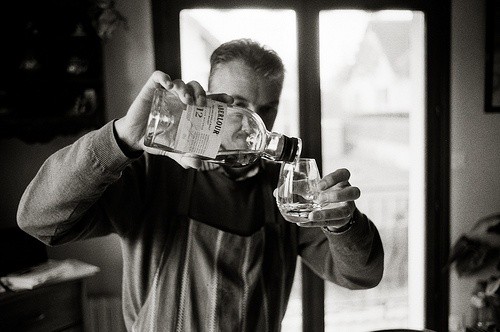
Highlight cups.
[276,159,323,223]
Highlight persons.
[17,39,385,332]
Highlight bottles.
[143,87,302,170]
